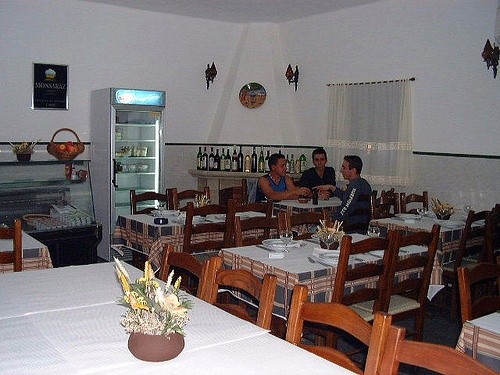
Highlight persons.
[298,149,336,199]
[316,155,372,235]
[256,153,308,205]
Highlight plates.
[435,219,466,228]
[151,208,174,215]
[318,252,339,264]
[239,83,266,109]
[262,238,299,251]
[395,213,421,220]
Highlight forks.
[308,257,329,267]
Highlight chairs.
[0,218,22,272]
[130,179,500,375]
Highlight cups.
[367,227,380,237]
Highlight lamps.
[482,39,500,79]
[204,62,217,90]
[285,64,299,92]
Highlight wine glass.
[279,230,293,253]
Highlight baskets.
[47,128,85,159]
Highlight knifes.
[256,245,275,252]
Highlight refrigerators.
[89,88,166,263]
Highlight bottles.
[196,145,308,174]
[312,188,318,205]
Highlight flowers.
[311,219,345,245]
[7,137,42,153]
[428,196,455,213]
[113,256,195,340]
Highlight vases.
[433,211,452,220]
[128,332,185,362]
[318,238,339,250]
[16,154,31,162]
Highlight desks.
[217,234,429,318]
[0,223,53,274]
[259,196,343,218]
[111,211,279,276]
[368,211,485,302]
[455,311,500,375]
[188,169,304,192]
[0,258,356,375]
